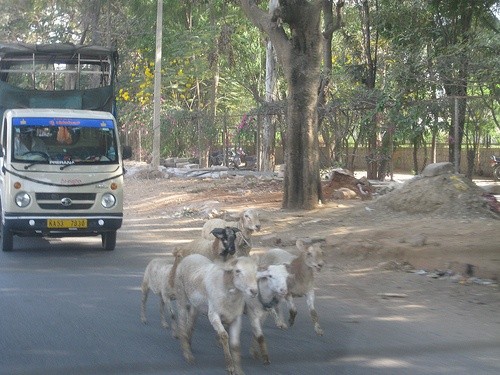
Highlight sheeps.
[173,252,261,375]
[171,224,243,265]
[245,263,297,366]
[255,235,327,339]
[138,244,198,337]
[200,205,262,257]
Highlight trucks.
[0,41,133,251]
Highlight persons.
[14,128,50,161]
[0,60,9,81]
